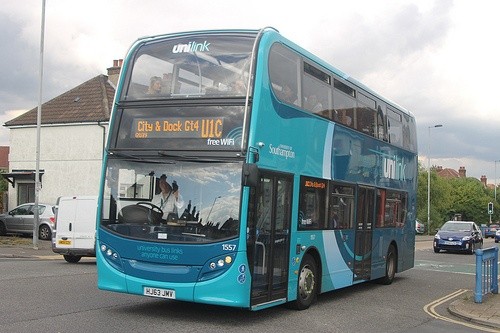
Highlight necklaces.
[159,189,171,209]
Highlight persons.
[334,100,352,126]
[148,174,184,220]
[307,91,323,115]
[281,80,298,105]
[146,76,162,93]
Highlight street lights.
[426,124,444,234]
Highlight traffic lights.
[487,202,493,214]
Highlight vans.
[51,197,120,263]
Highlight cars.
[0,203,55,241]
[415,219,425,234]
[485,226,497,239]
[495,230,500,243]
[479,226,487,237]
[433,221,484,255]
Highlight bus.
[96,28,418,312]
